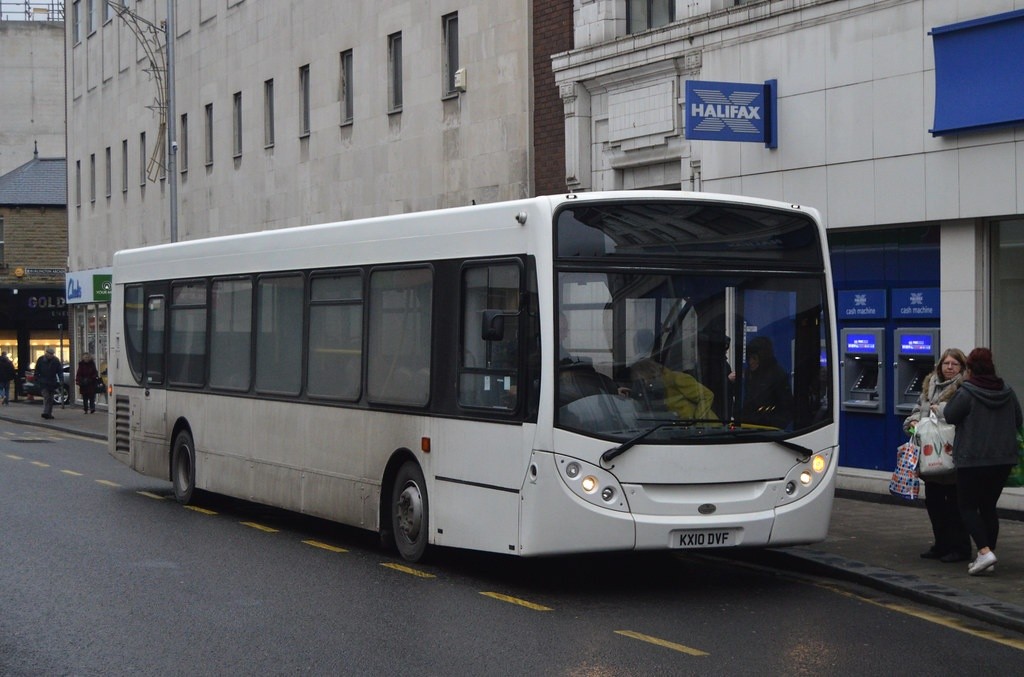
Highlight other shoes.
[41,413,54,419]
[939,552,971,562]
[91,410,95,413]
[920,550,946,558]
[1,396,8,405]
[84,411,88,414]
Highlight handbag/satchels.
[915,417,955,477]
[95,378,107,393]
[889,426,921,500]
[79,377,90,387]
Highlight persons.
[34,349,64,420]
[76,352,96,414]
[0,351,15,405]
[728,336,786,429]
[903,349,972,563]
[943,348,1024,576]
[630,359,724,427]
[505,309,633,398]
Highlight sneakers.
[968,551,998,576]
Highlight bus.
[107,191,844,563]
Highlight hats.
[46,348,55,354]
